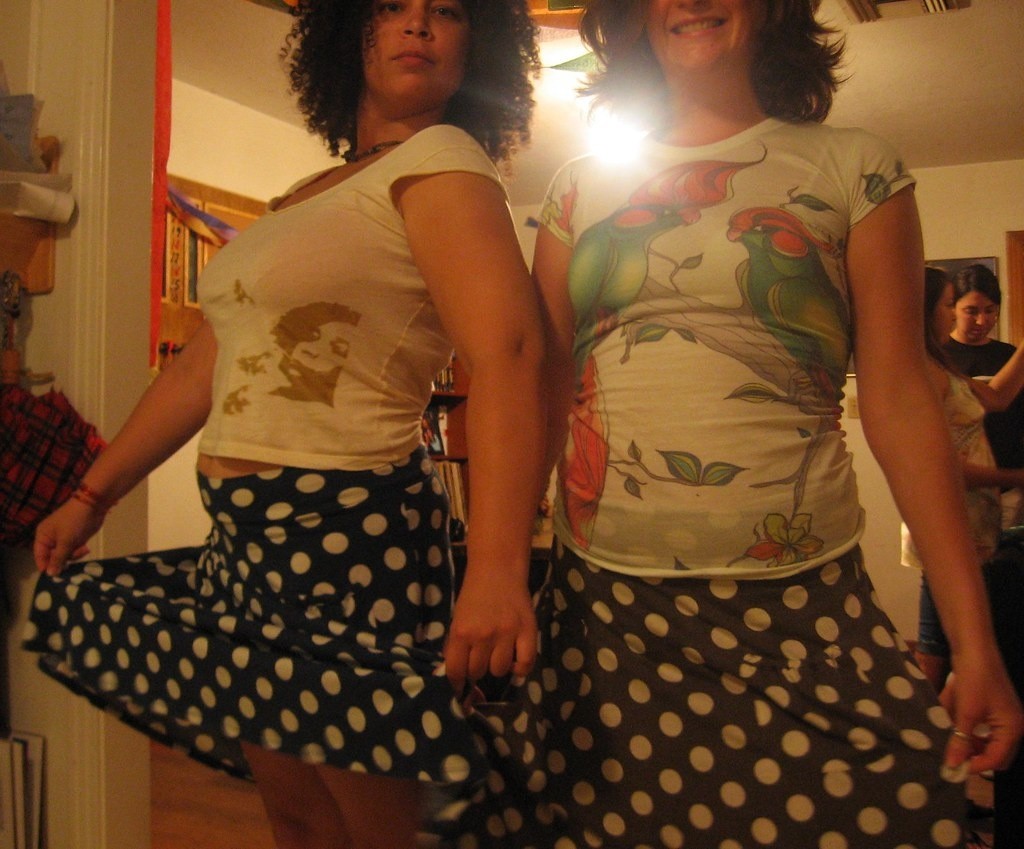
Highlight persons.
[535,0,1024,849]
[19,0,549,848]
[902,263,1024,693]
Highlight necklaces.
[342,141,402,160]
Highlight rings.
[952,729,968,739]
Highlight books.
[430,355,454,392]
[434,461,465,523]
[420,404,448,456]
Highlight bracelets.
[71,480,119,513]
